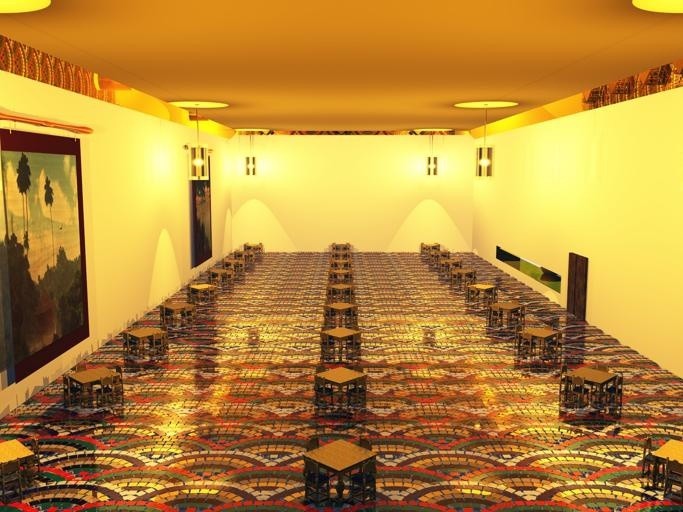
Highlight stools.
[304,239,377,512]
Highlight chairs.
[449,267,476,291]
[187,279,215,305]
[559,364,623,418]
[514,323,562,372]
[0,434,38,500]
[422,242,461,277]
[208,241,263,290]
[642,434,683,497]
[485,299,525,335]
[63,365,124,408]
[160,301,198,332]
[120,325,169,359]
[465,280,497,309]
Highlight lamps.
[192,102,207,178]
[476,105,493,178]
